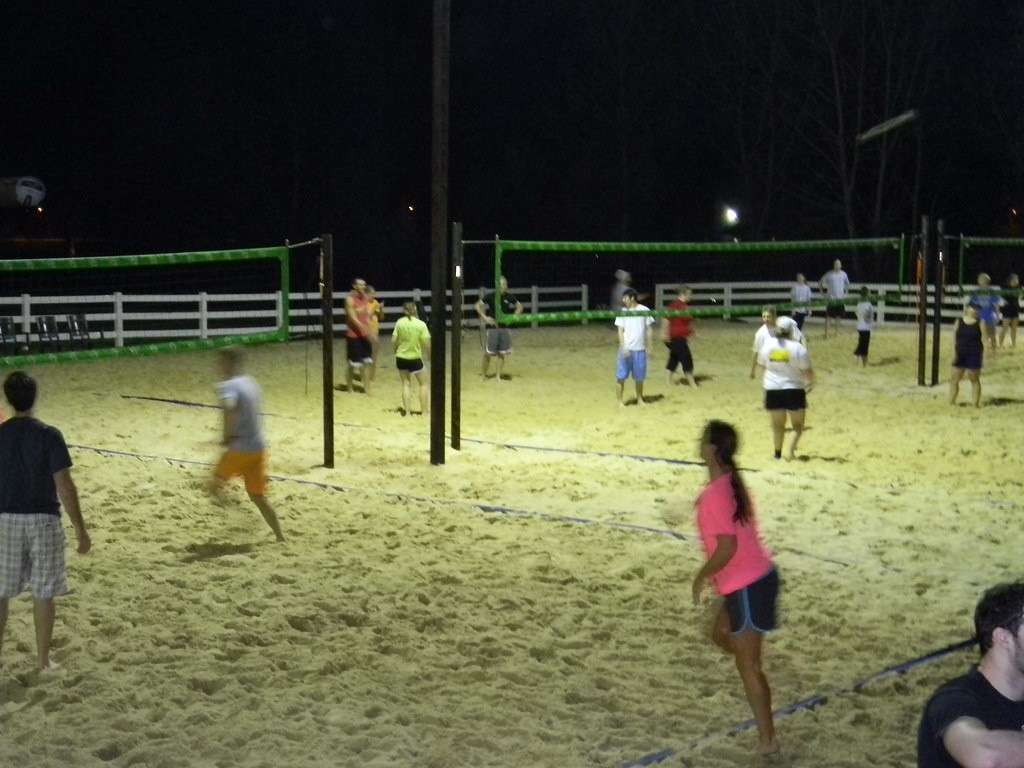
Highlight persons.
[971,272,998,355]
[916,581,1024,768]
[998,275,1024,348]
[748,305,814,458]
[692,418,780,756]
[391,301,431,416]
[790,274,813,328]
[207,344,288,545]
[612,270,650,309]
[949,301,988,407]
[614,289,655,408]
[0,372,91,670]
[661,286,697,388]
[817,259,850,338]
[474,276,523,380]
[854,287,873,368]
[344,279,384,395]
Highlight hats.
[968,300,982,309]
[775,316,797,330]
